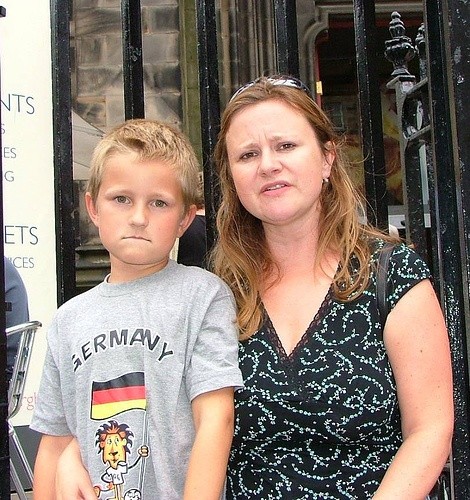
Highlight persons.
[28,120,243,499]
[204,74,453,500]
[5,256,29,386]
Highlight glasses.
[227,74,314,104]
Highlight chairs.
[3,320,42,500]
